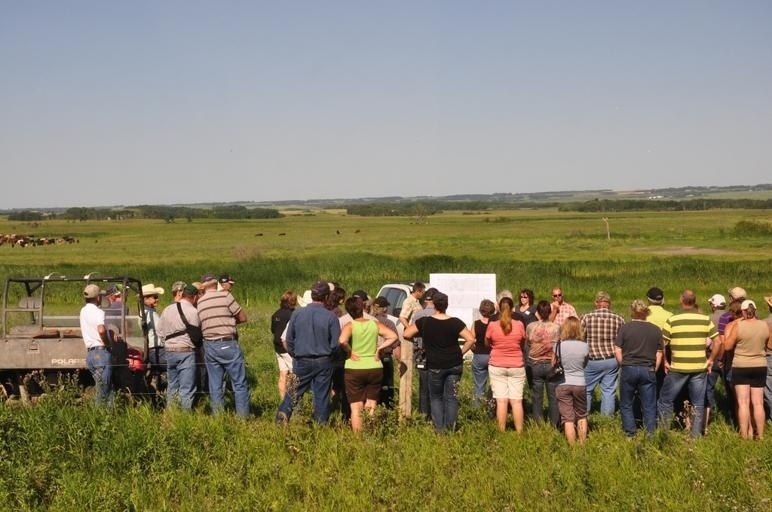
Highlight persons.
[134,270,251,420]
[400,282,476,434]
[77,283,117,415]
[106,283,130,317]
[269,281,401,434]
[469,287,772,444]
[98,285,116,307]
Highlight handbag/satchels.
[546,361,564,382]
[188,325,201,347]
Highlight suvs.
[1,274,151,406]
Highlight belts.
[207,338,232,341]
[165,348,192,351]
[87,346,105,351]
[589,355,614,360]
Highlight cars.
[377,284,475,362]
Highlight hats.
[647,287,772,310]
[297,281,391,308]
[84,274,236,298]
